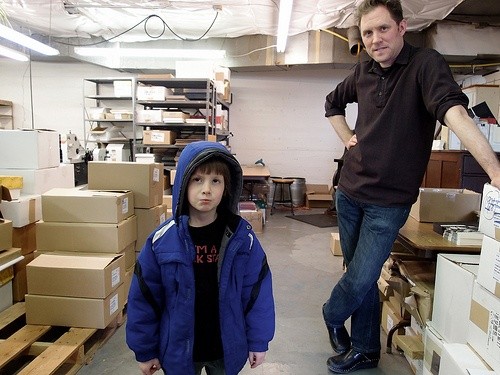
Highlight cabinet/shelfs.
[83,78,143,161]
[174,92,230,146]
[132,76,216,169]
[0,100,14,129]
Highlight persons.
[322,0,500,374]
[125,141,275,375]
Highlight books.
[435,223,483,246]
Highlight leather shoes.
[322,303,351,353]
[327,348,380,372]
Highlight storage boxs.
[135,60,231,124]
[0,126,176,330]
[208,134,216,142]
[331,182,500,375]
[86,80,133,120]
[441,121,500,152]
[239,201,265,232]
[306,184,333,208]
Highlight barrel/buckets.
[290,182,306,207]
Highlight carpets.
[285,213,338,228]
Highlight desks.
[386,214,481,354]
[240,165,270,209]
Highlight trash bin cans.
[285,177,306,207]
[268,177,283,201]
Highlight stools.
[270,179,295,216]
